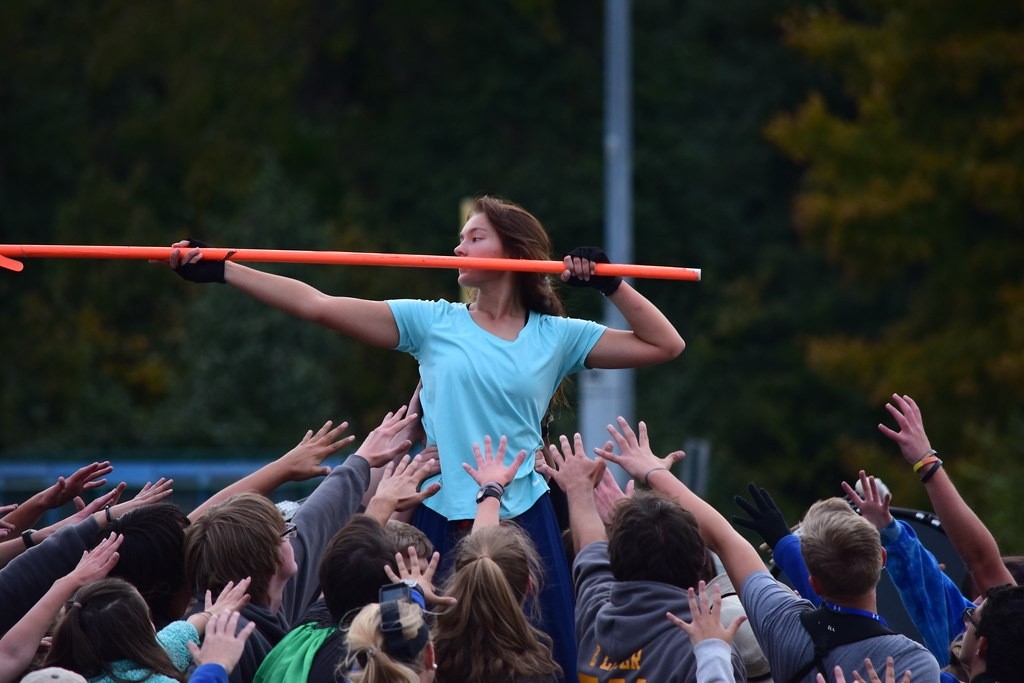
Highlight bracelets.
[646,467,667,486]
[913,450,943,483]
[198,610,212,620]
[105,504,111,521]
[22,529,38,548]
[485,482,505,496]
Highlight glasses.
[960,608,983,633]
[280,522,297,539]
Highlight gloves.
[731,482,793,551]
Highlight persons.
[0,379,1024,683]
[594,415,940,683]
[431,434,564,683]
[146,197,686,683]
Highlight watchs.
[399,579,425,597]
[476,487,501,504]
